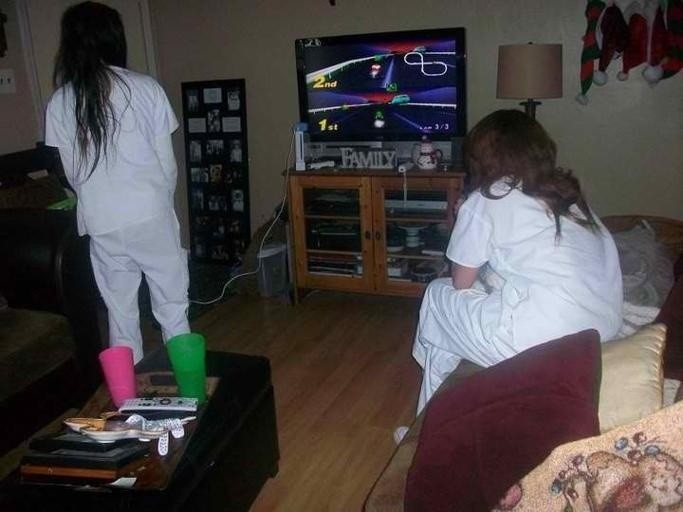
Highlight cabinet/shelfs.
[283,167,466,307]
[182,78,251,265]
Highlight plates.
[82,425,128,445]
[62,417,104,435]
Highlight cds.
[411,267,437,282]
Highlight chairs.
[0,216,102,455]
[0,140,77,216]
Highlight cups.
[166,332,209,407]
[98,346,139,407]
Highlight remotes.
[119,397,199,415]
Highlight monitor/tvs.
[295,26,466,142]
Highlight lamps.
[497,41,562,120]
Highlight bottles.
[291,122,311,166]
[417,140,438,171]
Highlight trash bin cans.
[257,240,288,299]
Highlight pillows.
[0,176,68,210]
[599,323,667,432]
[404,329,601,512]
[493,400,683,512]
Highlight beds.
[362,215,683,512]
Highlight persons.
[42,2,193,373]
[392,109,625,446]
[185,89,249,262]
